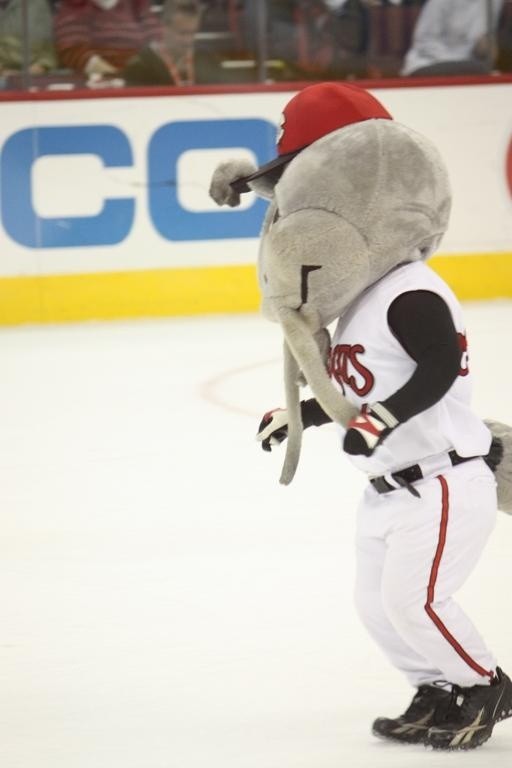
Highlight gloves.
[260,404,309,454]
[342,400,398,455]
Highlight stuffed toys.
[205,118,453,486]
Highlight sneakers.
[374,686,451,739]
[426,668,511,750]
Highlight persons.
[402,0,506,77]
[0,0,363,89]
[233,78,511,753]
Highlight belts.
[371,449,477,494]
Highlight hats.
[230,81,393,187]
